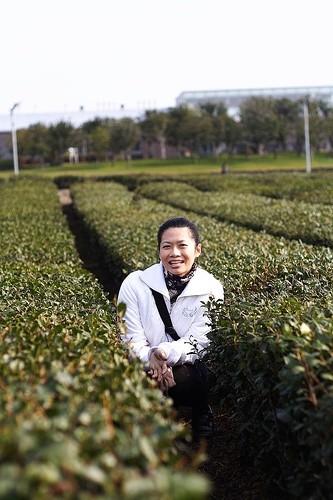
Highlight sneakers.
[190,404,215,440]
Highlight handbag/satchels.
[193,359,218,391]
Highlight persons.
[116,218,224,439]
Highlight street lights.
[300,89,317,174]
[8,101,22,175]
